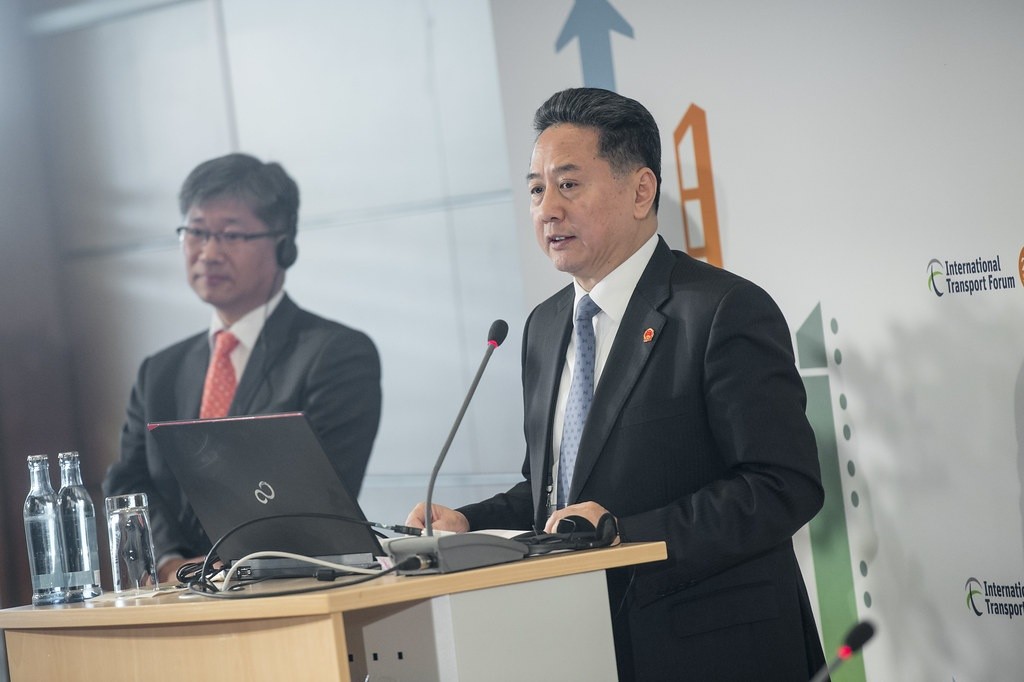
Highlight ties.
[199,331,240,418]
[556,294,601,510]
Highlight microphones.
[381,318,527,579]
[808,619,877,682]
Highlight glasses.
[176,226,285,256]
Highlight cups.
[104,493,159,597]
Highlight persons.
[404,88,833,682]
[100,152,380,586]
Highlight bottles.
[57,451,103,602]
[23,454,70,607]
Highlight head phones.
[264,168,299,269]
[517,513,618,555]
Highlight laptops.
[150,413,403,579]
